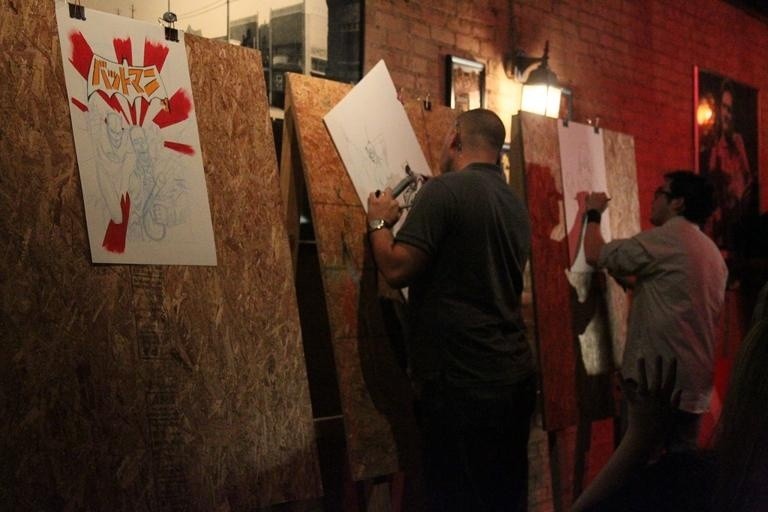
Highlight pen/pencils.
[606,198,612,201]
[398,204,414,210]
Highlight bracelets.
[587,210,600,225]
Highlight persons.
[710,90,754,209]
[582,169,731,452]
[567,311,767,511]
[364,108,541,508]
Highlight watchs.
[366,217,390,233]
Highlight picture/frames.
[693,65,760,201]
[445,55,485,112]
[560,86,574,121]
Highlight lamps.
[514,39,562,119]
